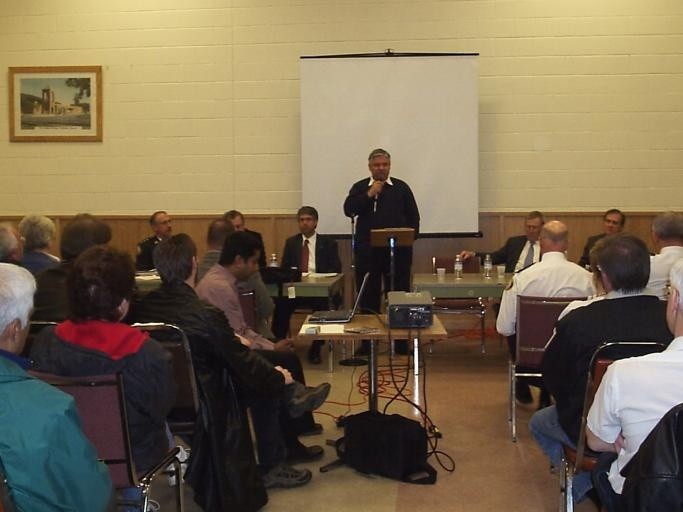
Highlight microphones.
[372,176,384,215]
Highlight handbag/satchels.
[333,410,427,479]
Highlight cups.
[435,268,445,276]
[497,265,506,278]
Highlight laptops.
[308,272,370,323]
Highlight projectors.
[387,291,433,328]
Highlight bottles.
[483,254,492,279]
[453,255,462,279]
[268,254,277,267]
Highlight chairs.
[502,296,682,510]
[130,323,258,463]
[430,254,487,358]
[20,322,58,357]
[33,372,183,512]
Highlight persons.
[343,149,420,355]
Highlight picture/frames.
[6,64,102,144]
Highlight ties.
[301,241,308,272]
[524,241,536,269]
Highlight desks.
[297,307,446,409]
[131,267,344,361]
[411,270,518,362]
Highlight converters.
[429,425,441,438]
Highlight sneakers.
[515,383,533,405]
[165,447,190,486]
[288,382,331,416]
[258,464,312,490]
[299,424,322,437]
[282,445,324,464]
